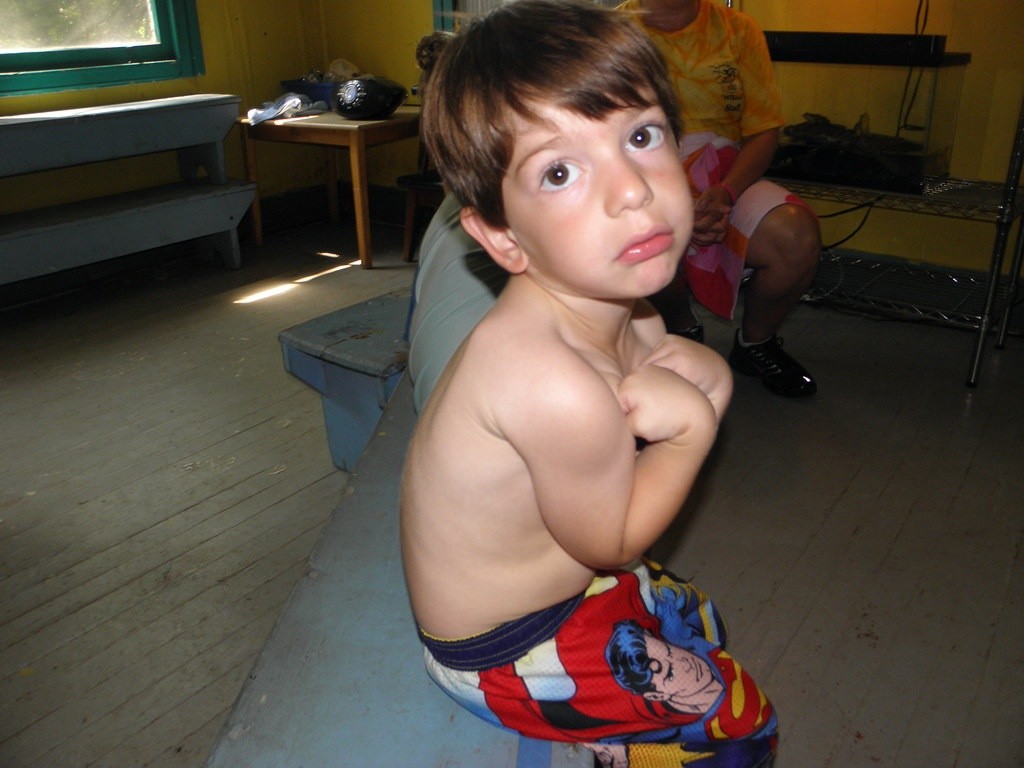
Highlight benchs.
[0,93,262,288]
[205,358,595,768]
[277,282,416,474]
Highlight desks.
[236,105,421,268]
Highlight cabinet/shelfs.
[762,99,1024,389]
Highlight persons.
[396,0,782,768]
[603,0,823,398]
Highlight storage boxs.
[280,79,340,111]
[769,50,972,176]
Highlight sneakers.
[650,284,706,343]
[730,328,817,397]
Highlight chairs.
[396,84,446,263]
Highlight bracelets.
[717,179,738,204]
